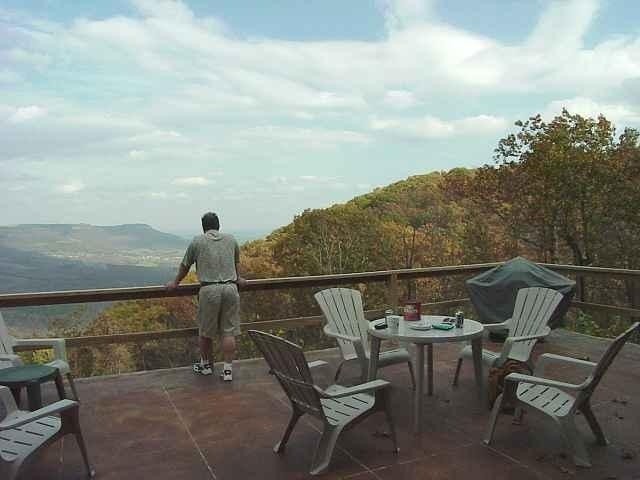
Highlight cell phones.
[375,322,387,329]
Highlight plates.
[408,321,432,330]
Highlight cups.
[387,315,401,337]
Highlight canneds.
[384,309,394,327]
[456,313,462,327]
[459,311,465,323]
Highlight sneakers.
[193,363,213,376]
[221,369,233,381]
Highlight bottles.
[384,310,392,326]
[457,312,464,328]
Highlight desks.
[367,315,486,438]
[0,364,68,413]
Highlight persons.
[167,212,243,381]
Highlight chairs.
[481,321,640,469]
[0,383,98,480]
[0,314,82,402]
[451,287,565,419]
[312,287,415,391]
[246,328,402,477]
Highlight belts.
[200,281,238,286]
